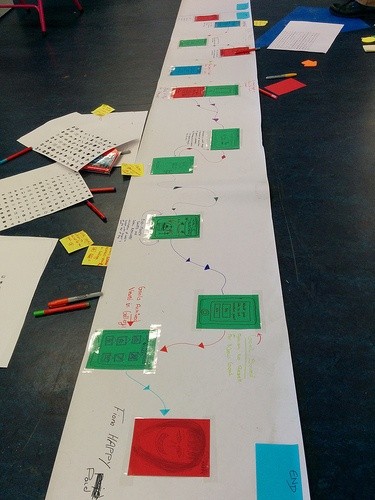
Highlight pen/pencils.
[266,73,297,79]
[49,292,103,308]
[120,150,131,154]
[234,47,260,53]
[89,187,116,191]
[33,303,89,316]
[259,88,276,98]
[0,146,32,164]
[87,199,106,220]
